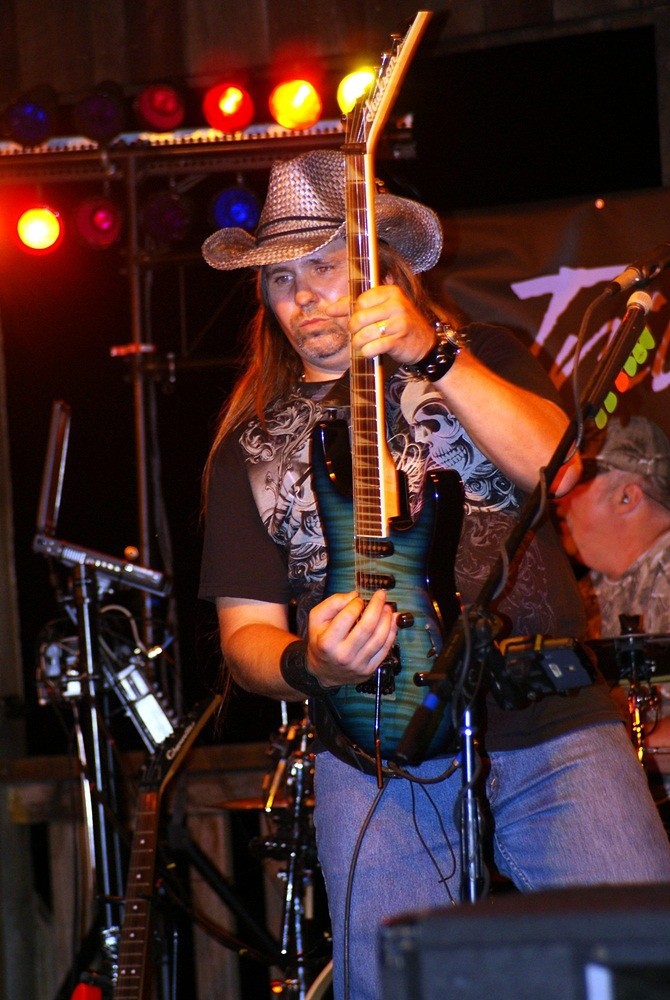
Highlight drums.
[304,959,334,1000]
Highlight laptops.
[105,667,275,773]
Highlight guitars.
[310,32,466,767]
[72,713,197,1000]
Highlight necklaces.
[300,369,307,382]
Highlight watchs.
[406,322,464,381]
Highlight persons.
[195,151,670,1000]
[555,417,670,818]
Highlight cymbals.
[207,794,316,813]
[585,633,670,686]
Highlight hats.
[202,149,443,276]
[579,413,670,483]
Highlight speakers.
[9,142,403,757]
[377,874,670,1000]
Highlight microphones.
[605,238,670,298]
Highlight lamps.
[211,176,262,235]
[16,203,66,254]
[140,80,193,130]
[4,86,63,143]
[78,80,128,138]
[79,193,124,246]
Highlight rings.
[377,321,387,335]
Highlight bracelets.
[281,639,340,700]
